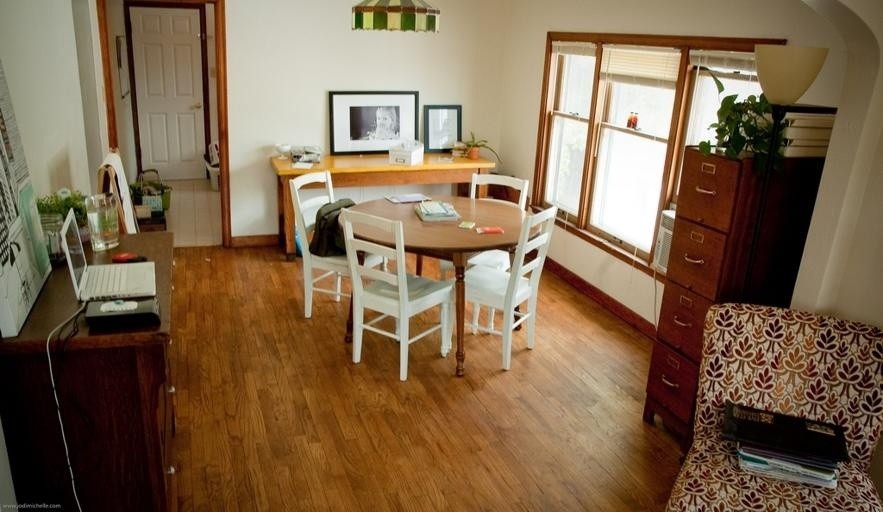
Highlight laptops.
[59,208,157,302]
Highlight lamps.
[742,43,829,305]
[351,1,441,32]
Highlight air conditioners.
[651,209,676,277]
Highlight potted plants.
[698,70,795,165]
[462,130,502,165]
[36,190,91,242]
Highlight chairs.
[440,173,530,322]
[448,205,558,370]
[664,303,882,512]
[340,208,455,381]
[287,169,389,319]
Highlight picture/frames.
[424,105,462,154]
[328,92,419,155]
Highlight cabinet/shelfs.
[637,145,824,452]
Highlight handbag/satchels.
[309,198,356,256]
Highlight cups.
[292,146,305,163]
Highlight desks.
[338,194,541,377]
[0,232,176,512]
[270,153,495,262]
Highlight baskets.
[129,168,172,212]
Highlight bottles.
[38,213,64,268]
[85,192,119,252]
[627,112,639,128]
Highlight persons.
[362,106,397,140]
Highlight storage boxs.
[206,161,220,191]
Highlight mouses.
[111,252,148,263]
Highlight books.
[736,441,843,489]
[414,199,460,222]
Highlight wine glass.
[274,144,291,160]
